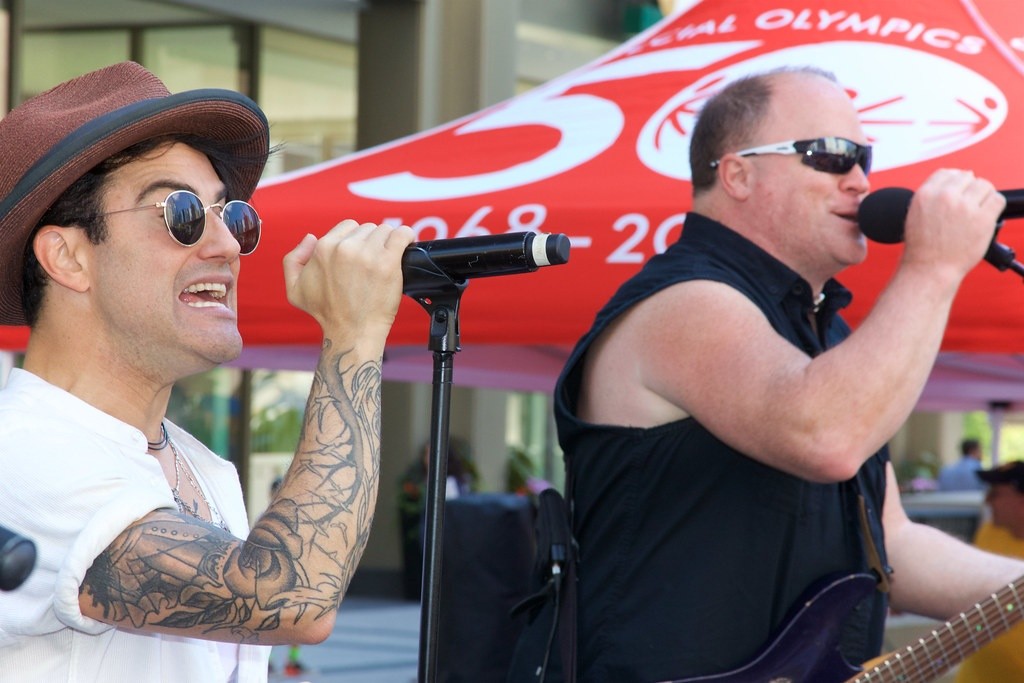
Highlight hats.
[974,460,1024,489]
[1,60,270,326]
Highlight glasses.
[80,189,262,255]
[709,136,873,175]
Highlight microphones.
[858,187,1024,243]
[400,231,570,292]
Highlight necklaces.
[147,421,229,531]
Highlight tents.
[0,0,1024,465]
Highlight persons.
[553,68,1024,683]
[0,61,418,683]
[976,459,1024,541]
[938,438,986,490]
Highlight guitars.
[650,563,1024,683]
